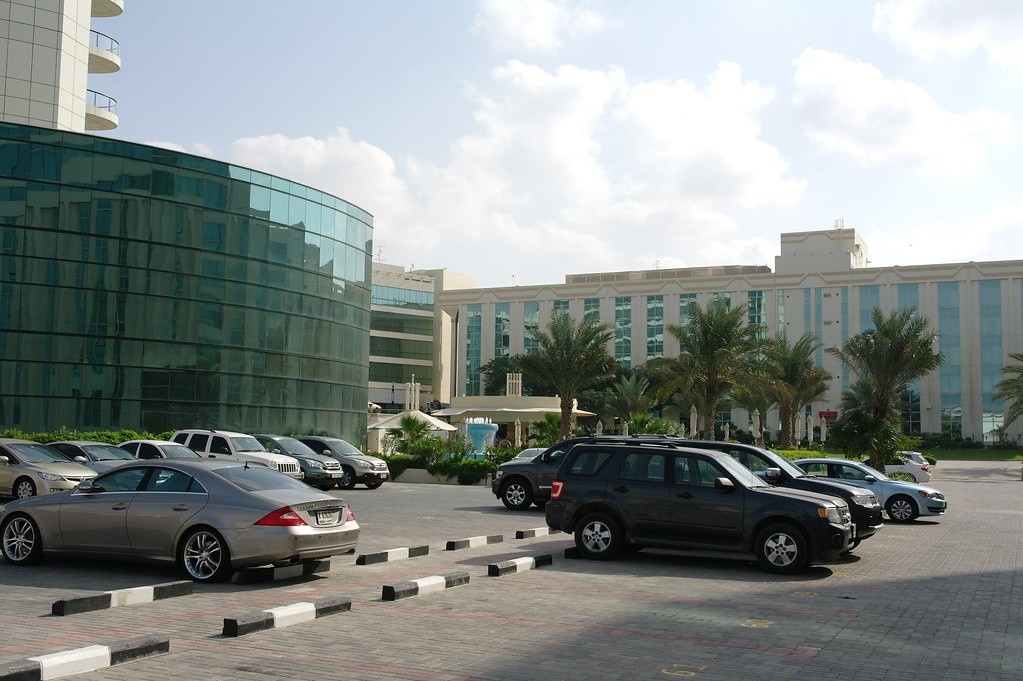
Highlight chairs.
[631,463,649,478]
[620,460,631,477]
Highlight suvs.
[620,434,885,549]
[252,434,344,492]
[490,434,632,512]
[168,429,301,481]
[289,435,391,490]
[545,440,860,577]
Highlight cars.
[0,438,98,501]
[840,455,932,484]
[47,441,171,486]
[118,440,203,461]
[0,458,361,584]
[754,458,948,524]
[514,447,562,462]
[896,451,930,469]
